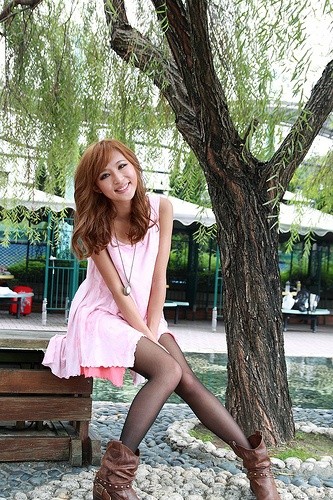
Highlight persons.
[42,139,279,500]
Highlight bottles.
[297,281,301,292]
[285,281,290,292]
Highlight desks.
[282,292,297,296]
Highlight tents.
[0,182,333,245]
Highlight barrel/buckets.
[11,285,33,315]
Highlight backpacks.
[291,290,311,311]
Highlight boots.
[228,430,281,500]
[93,440,140,500]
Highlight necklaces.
[111,219,137,296]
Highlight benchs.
[1,329,101,466]
[164,301,189,324]
[0,287,34,318]
[281,309,330,333]
